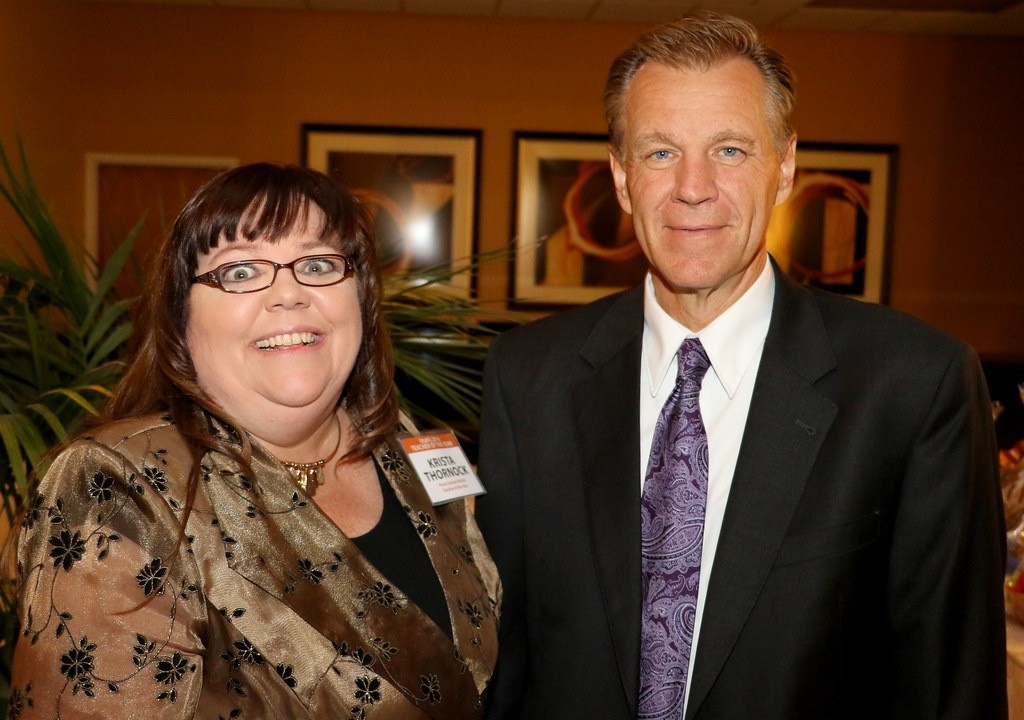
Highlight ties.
[638,337,712,720]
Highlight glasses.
[190,254,356,294]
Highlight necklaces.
[279,412,341,496]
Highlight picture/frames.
[85,151,239,314]
[299,122,481,308]
[764,140,898,307]
[508,128,639,312]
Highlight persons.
[1,160,503,720]
[476,10,1008,720]
